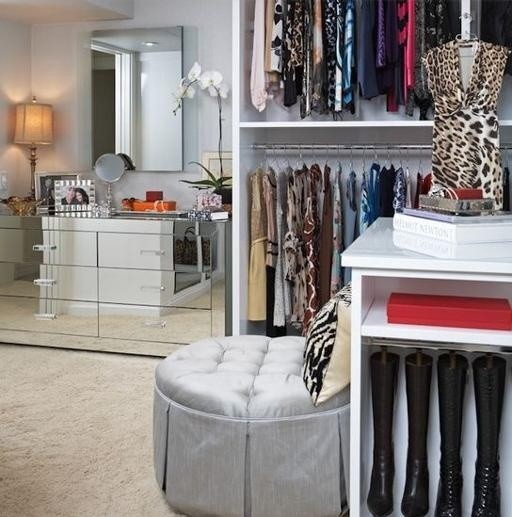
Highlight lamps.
[12,96,54,201]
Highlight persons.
[75,188,88,205]
[61,187,82,205]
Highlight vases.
[212,190,232,204]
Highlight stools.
[151,333,349,517]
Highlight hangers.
[253,140,512,186]
[416,11,509,62]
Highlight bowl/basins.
[0,196,46,216]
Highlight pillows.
[299,280,353,408]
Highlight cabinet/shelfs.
[33,214,229,317]
[0,216,42,266]
[231,0,512,335]
[339,215,512,517]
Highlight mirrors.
[90,25,185,173]
[94,153,125,217]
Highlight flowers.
[165,60,231,191]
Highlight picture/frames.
[54,180,96,217]
[200,151,233,189]
[33,171,82,213]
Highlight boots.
[435,353,469,516]
[401,352,434,517]
[471,356,507,516]
[367,351,400,517]
